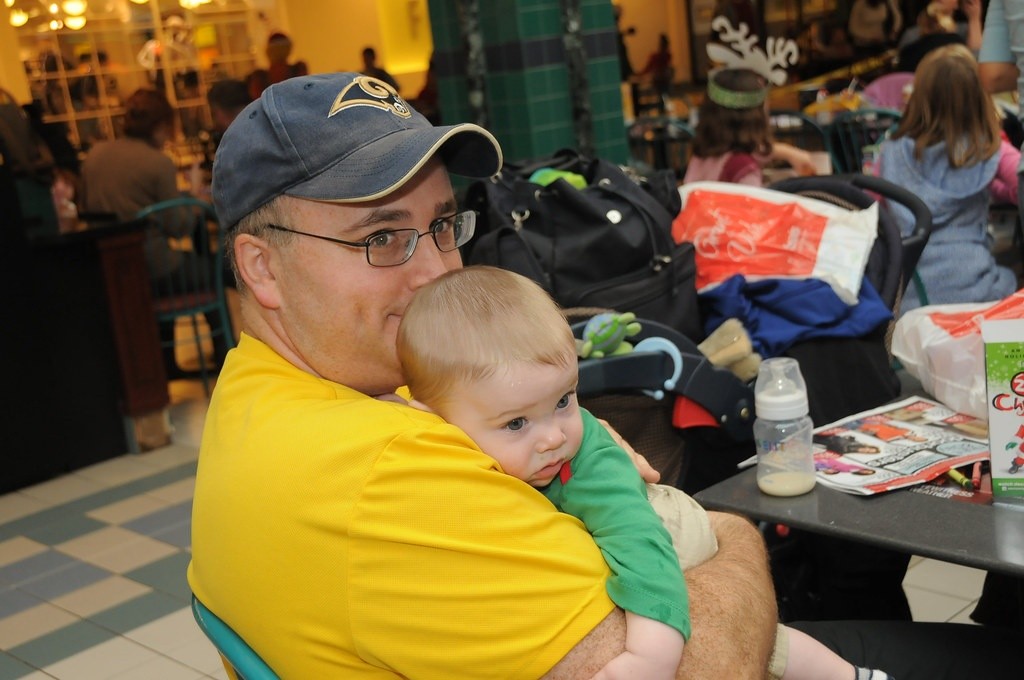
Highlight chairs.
[190,75,1024,680]
[137,197,235,398]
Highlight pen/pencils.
[948,468,974,489]
[971,460,981,486]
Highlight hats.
[211,70,503,230]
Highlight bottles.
[752,358,817,497]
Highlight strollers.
[569,175,932,624]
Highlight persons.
[188,70,1024,680]
[0,29,400,379]
[614,4,635,81]
[842,419,928,442]
[849,0,1024,318]
[682,67,818,188]
[815,459,875,475]
[812,435,880,454]
[396,265,895,680]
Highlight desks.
[694,462,1024,625]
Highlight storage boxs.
[983,318,1024,496]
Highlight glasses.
[260,211,481,272]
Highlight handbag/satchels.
[469,146,705,348]
